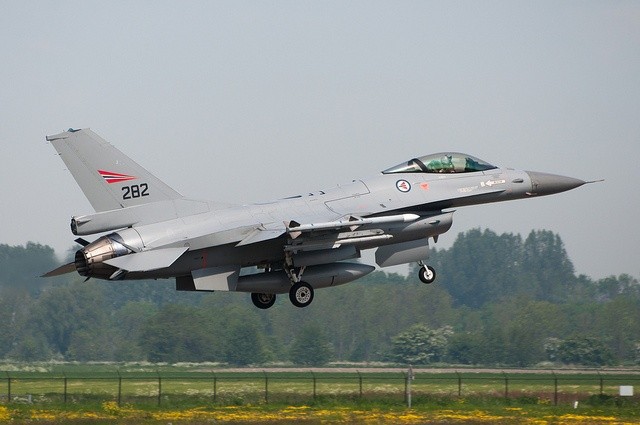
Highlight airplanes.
[39,127,606,308]
